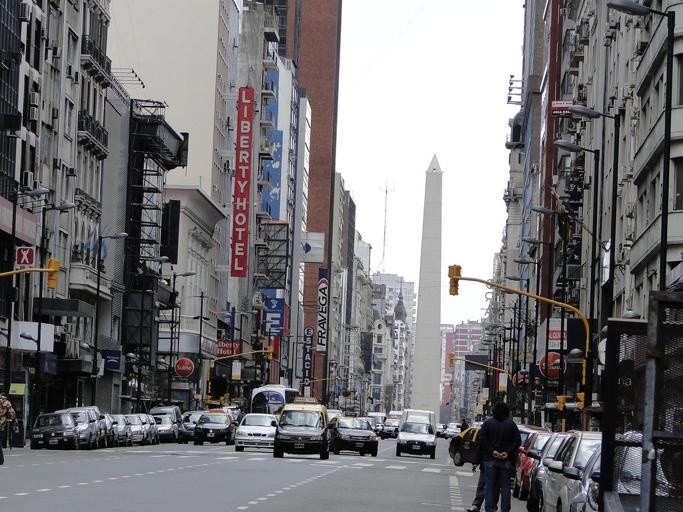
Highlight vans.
[272,395,331,460]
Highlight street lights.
[209,302,259,402]
[19,201,78,408]
[554,134,608,432]
[80,231,129,405]
[477,200,569,422]
[159,265,195,404]
[118,253,170,412]
[189,289,212,358]
[606,0,666,433]
[124,252,168,413]
[1,186,51,397]
[565,100,626,435]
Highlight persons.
[461,419,469,432]
[466,446,500,512]
[237,408,246,424]
[0,389,16,465]
[479,401,521,512]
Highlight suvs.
[326,407,346,422]
[182,411,206,444]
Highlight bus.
[251,384,299,416]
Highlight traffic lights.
[207,395,212,404]
[220,397,224,404]
[575,391,584,410]
[556,394,562,409]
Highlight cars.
[193,409,236,446]
[353,410,481,469]
[274,414,281,423]
[236,412,278,452]
[330,414,380,458]
[29,403,182,450]
[509,418,681,512]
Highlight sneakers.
[466,505,480,512]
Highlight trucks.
[221,406,241,420]
